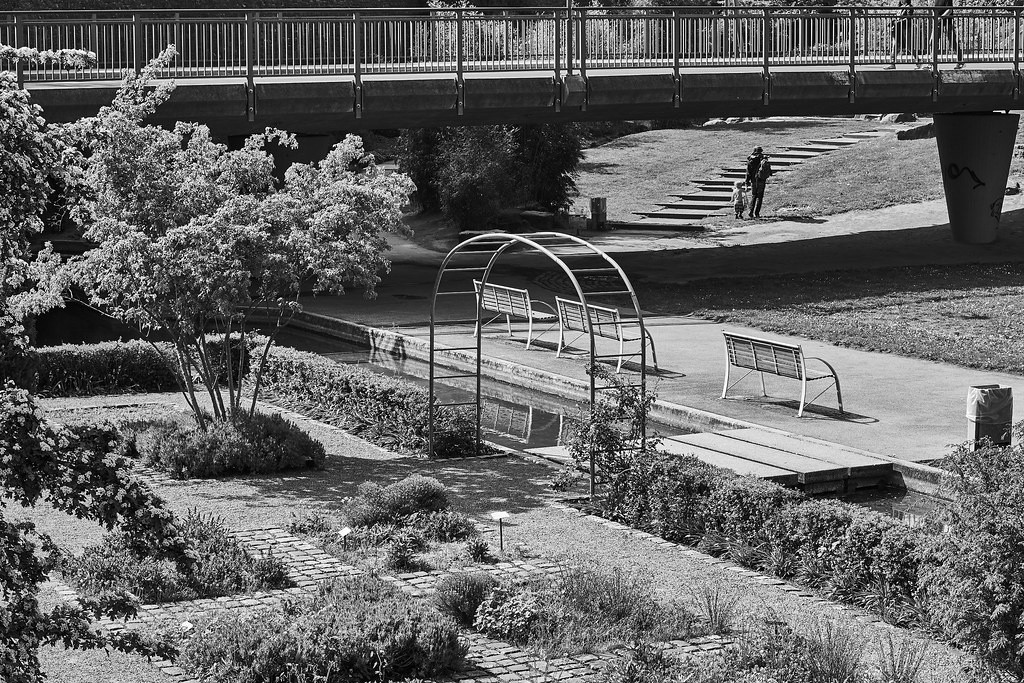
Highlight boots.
[739,212,743,218]
[735,214,739,219]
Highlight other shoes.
[756,215,762,218]
[748,212,753,217]
[886,64,896,69]
[922,64,932,69]
[915,64,921,69]
[953,63,966,69]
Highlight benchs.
[473,279,565,350]
[554,296,658,374]
[721,329,844,418]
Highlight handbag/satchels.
[755,155,770,181]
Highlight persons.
[744,146,767,218]
[729,181,747,219]
[882,0,924,70]
[922,0,965,70]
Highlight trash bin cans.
[590,197,607,222]
[968,384,1013,452]
